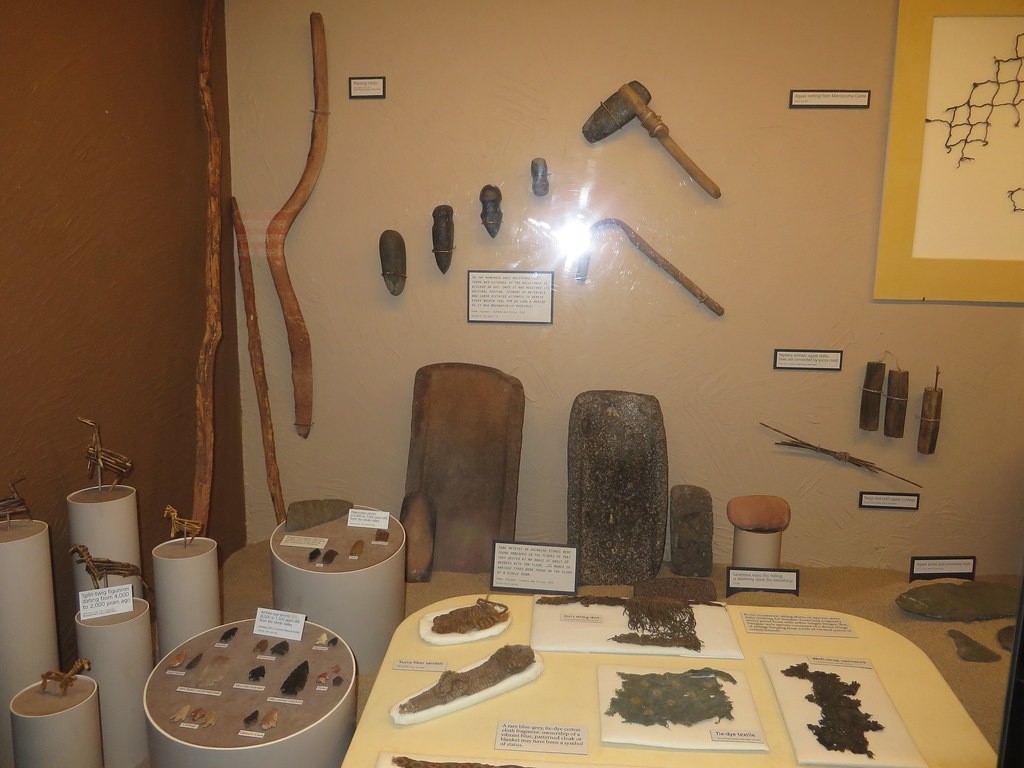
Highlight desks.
[339,592,997,768]
